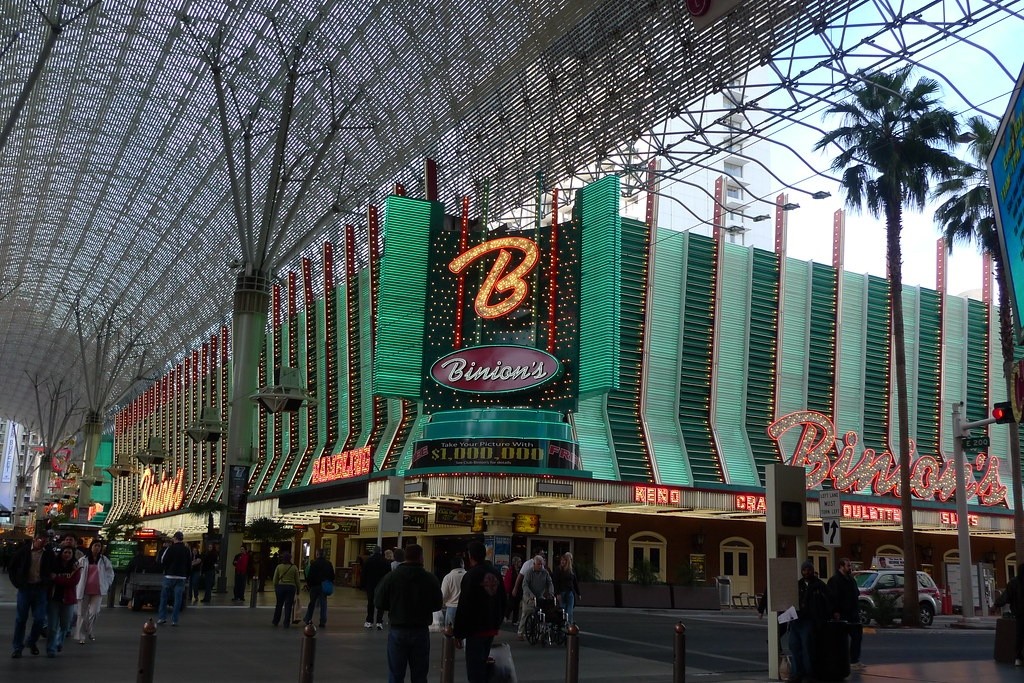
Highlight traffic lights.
[992,402,1016,425]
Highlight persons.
[375,544,442,683]
[304,548,578,641]
[989,563,1024,667]
[455,541,505,683]
[2,529,250,659]
[758,556,866,683]
[302,548,335,628]
[273,551,301,629]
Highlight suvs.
[849,569,942,626]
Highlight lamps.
[925,542,935,559]
[694,527,705,551]
[988,545,997,562]
[15,390,305,516]
[851,535,862,558]
[637,61,979,236]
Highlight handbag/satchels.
[322,580,333,596]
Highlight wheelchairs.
[525,594,569,648]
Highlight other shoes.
[849,661,867,669]
[318,623,325,628]
[157,618,168,624]
[284,623,289,628]
[11,627,96,658]
[192,595,245,602]
[513,622,519,625]
[503,616,508,624]
[516,635,524,641]
[376,623,384,630]
[172,620,177,626]
[1015,659,1021,666]
[302,619,310,625]
[272,622,277,627]
[364,621,372,628]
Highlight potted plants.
[616,560,671,608]
[573,562,615,606]
[670,566,720,610]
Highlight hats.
[172,532,183,539]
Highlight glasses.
[38,538,50,545]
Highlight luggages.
[821,617,851,683]
[993,609,1017,663]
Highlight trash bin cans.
[820,621,850,678]
[712,576,732,609]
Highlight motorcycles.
[118,569,189,613]
